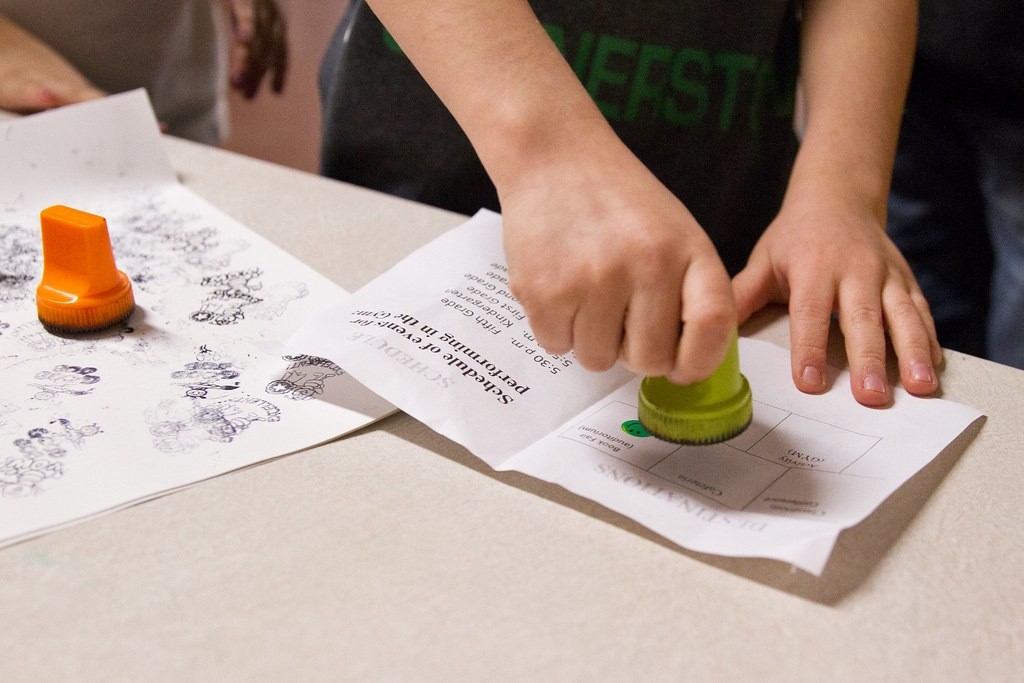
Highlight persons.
[316,0,997,407]
[0,0,288,150]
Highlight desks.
[0,110,1024,683]
